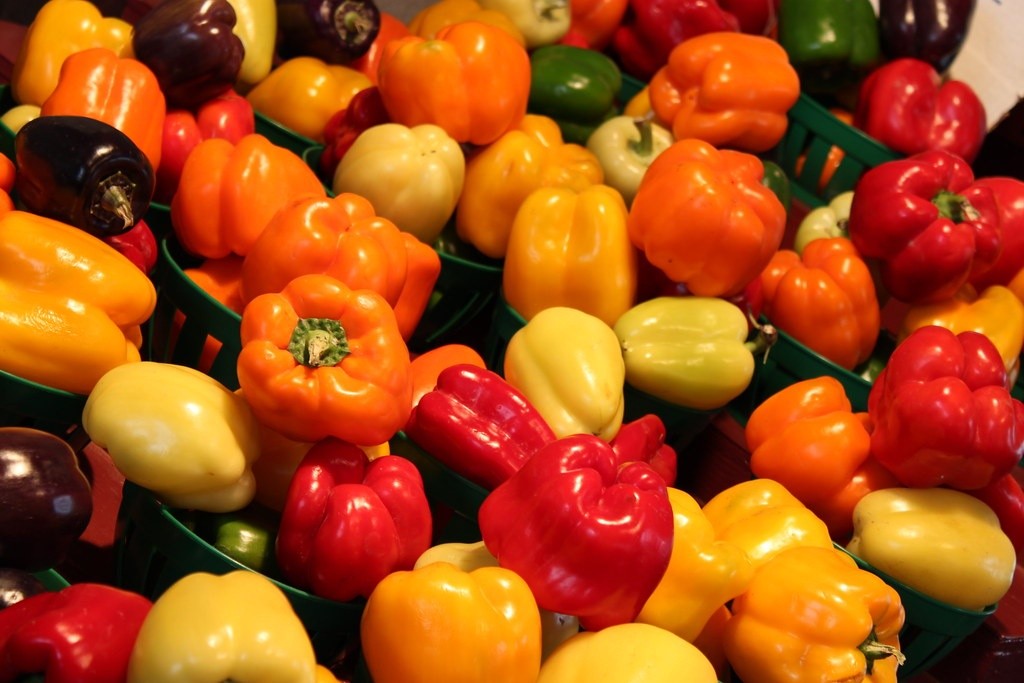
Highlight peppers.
[0,0,1024,682]
